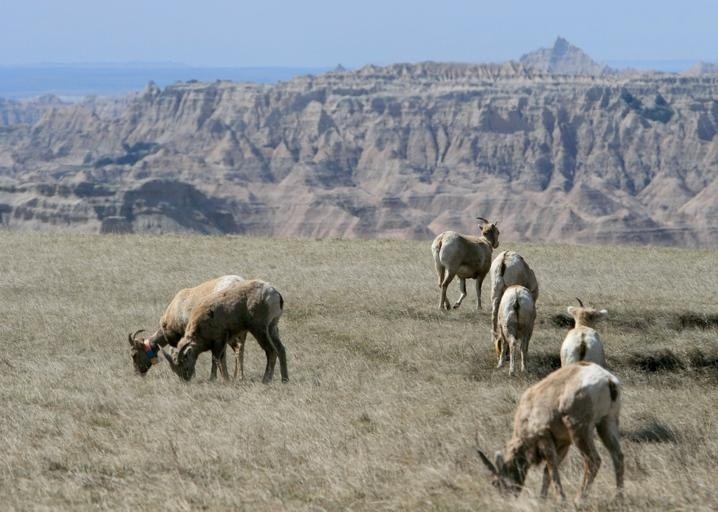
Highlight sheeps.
[431,216,500,312]
[560,297,608,369]
[128,274,290,386]
[489,251,539,376]
[478,360,625,505]
